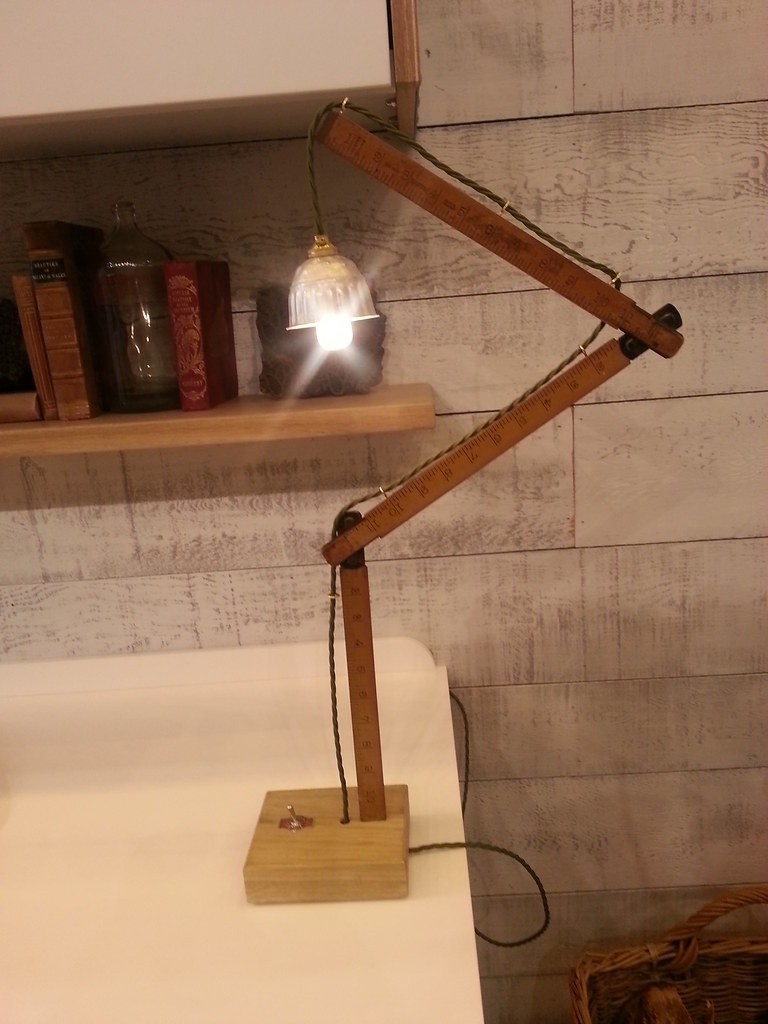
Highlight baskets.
[570,886,768,1024]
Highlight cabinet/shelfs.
[0,0,423,165]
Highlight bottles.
[90,201,181,412]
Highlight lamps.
[241,100,686,908]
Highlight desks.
[0,637,485,1023]
